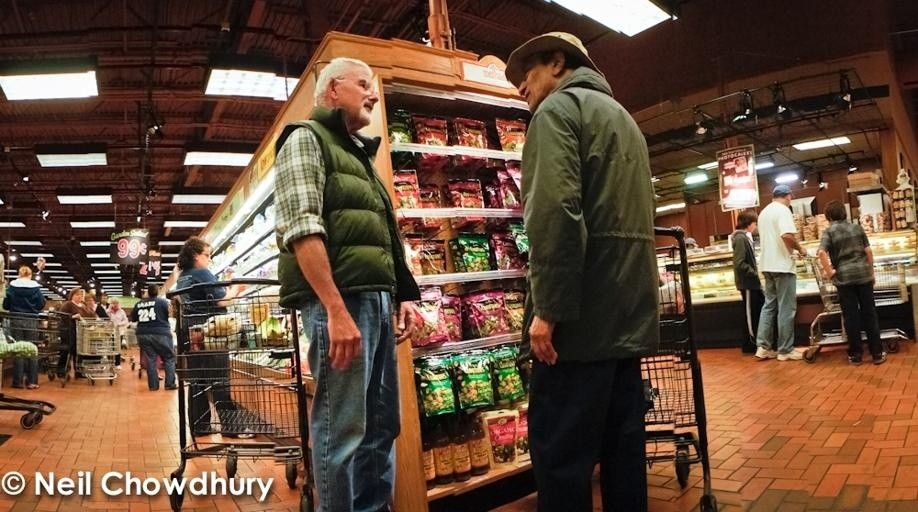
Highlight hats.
[505,32,605,89]
[773,185,791,195]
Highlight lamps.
[677,89,859,192]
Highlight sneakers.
[848,354,862,364]
[159,376,179,389]
[27,383,39,388]
[742,343,802,361]
[194,426,255,438]
[873,352,887,364]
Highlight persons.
[503,29,664,512]
[272,55,427,512]
[753,183,811,363]
[38,286,179,391]
[176,239,260,441]
[2,266,46,391]
[818,198,887,365]
[729,210,767,354]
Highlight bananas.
[257,317,293,345]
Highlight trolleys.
[791,245,912,364]
[631,225,721,512]
